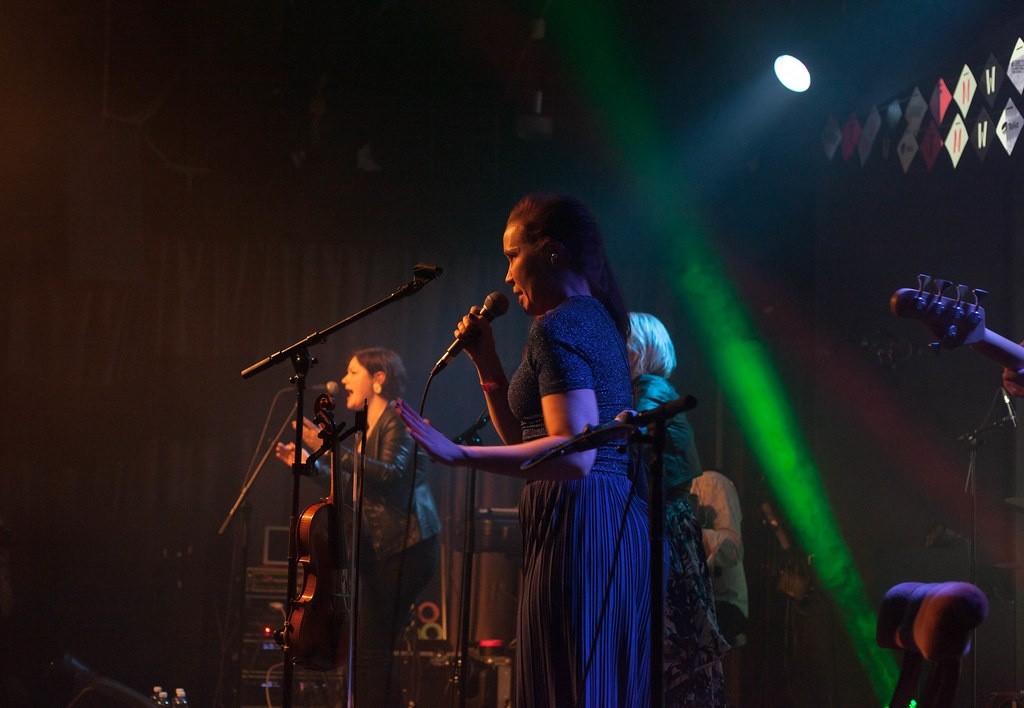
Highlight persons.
[274,346,446,708]
[621,305,727,708]
[687,469,750,653]
[389,190,651,708]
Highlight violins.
[283,393,358,669]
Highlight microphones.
[1002,388,1018,428]
[763,503,790,549]
[286,382,339,395]
[530,410,640,464]
[434,291,509,377]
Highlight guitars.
[888,274,1024,376]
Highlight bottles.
[172,688,188,708]
[157,692,172,708]
[151,687,162,704]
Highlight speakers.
[58,670,160,708]
